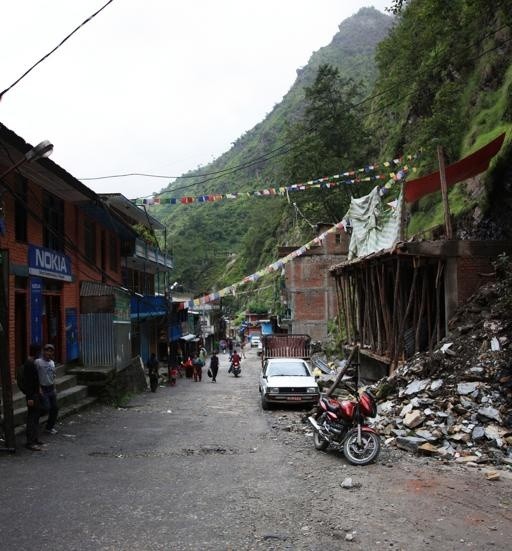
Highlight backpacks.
[16,358,33,394]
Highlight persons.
[17,343,45,450]
[34,344,59,434]
[170,337,247,385]
[147,353,159,392]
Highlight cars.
[257,356,320,409]
[250,335,261,347]
[257,341,265,354]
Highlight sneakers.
[26,427,58,452]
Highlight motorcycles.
[304,388,384,465]
[228,357,241,377]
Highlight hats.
[44,344,55,351]
